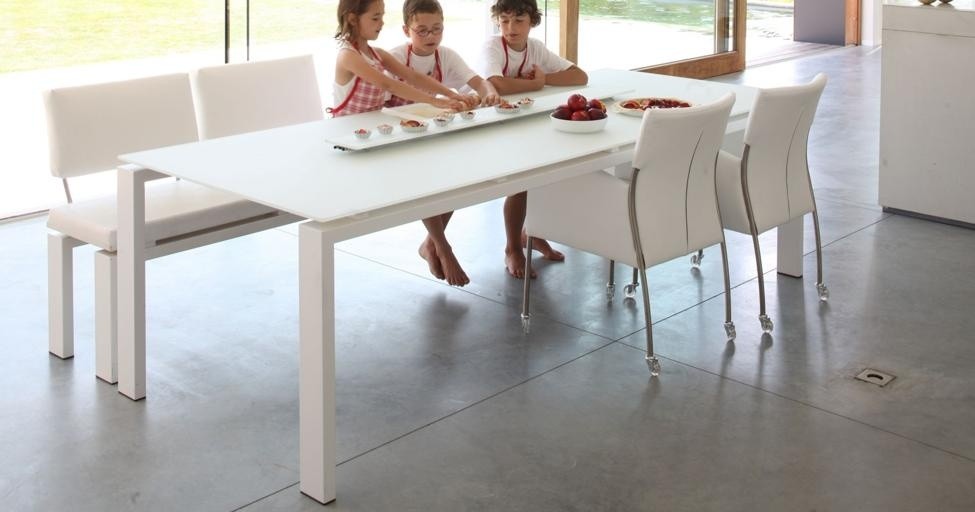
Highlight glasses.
[404,19,445,37]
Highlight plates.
[620,98,692,117]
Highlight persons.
[383,1,506,287]
[326,1,477,119]
[478,1,590,282]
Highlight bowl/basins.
[551,111,610,132]
[495,99,534,112]
[433,118,453,128]
[460,111,476,121]
[402,123,426,133]
[376,126,390,136]
[355,131,371,140]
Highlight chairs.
[519,91,737,378]
[622,71,830,332]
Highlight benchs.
[43,53,332,384]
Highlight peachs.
[554,94,606,121]
